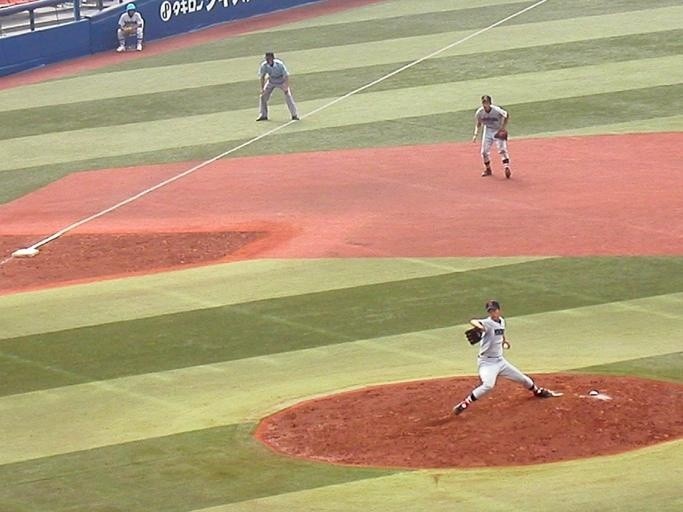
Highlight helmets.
[127,4,135,10]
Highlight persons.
[453,300,553,415]
[255,52,301,121]
[116,3,145,52]
[471,95,511,178]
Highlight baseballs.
[504,343,508,349]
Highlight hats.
[482,96,491,103]
[485,300,499,312]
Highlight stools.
[126,34,137,51]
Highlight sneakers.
[292,116,299,120]
[482,168,492,176]
[505,167,511,177]
[256,117,267,121]
[534,387,553,397]
[453,402,466,414]
[117,46,125,52]
[136,44,142,50]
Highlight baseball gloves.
[465,322,482,344]
[494,129,507,139]
